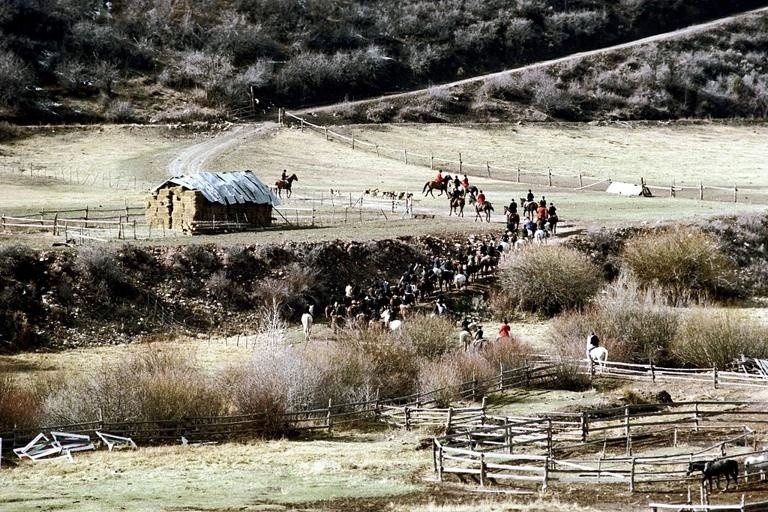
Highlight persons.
[500,188,557,246]
[435,170,486,210]
[303,235,510,341]
[588,332,599,353]
[282,170,289,183]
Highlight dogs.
[364,188,414,201]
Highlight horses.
[496,332,515,343]
[501,197,559,253]
[299,303,315,336]
[322,231,502,352]
[446,179,494,223]
[685,458,740,494]
[273,174,298,198]
[422,175,453,199]
[584,334,609,373]
